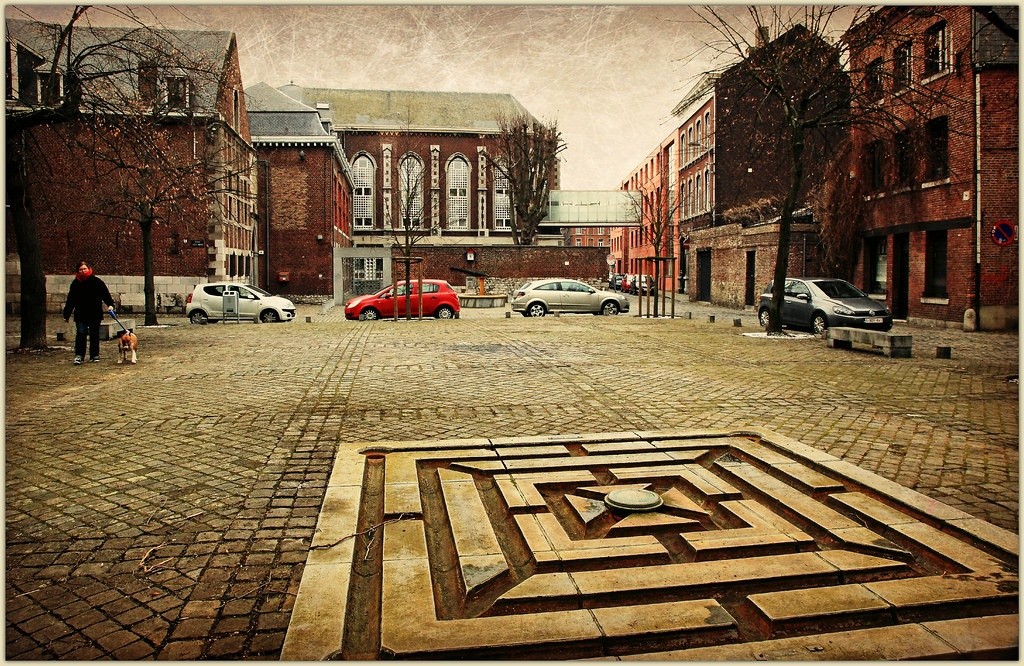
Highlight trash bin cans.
[466,277,476,293]
[223,291,239,324]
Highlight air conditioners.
[478,229,489,237]
[431,227,441,236]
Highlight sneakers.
[89,355,100,363]
[73,355,84,365]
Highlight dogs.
[117,332,138,363]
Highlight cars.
[510,278,630,316]
[185,281,297,324]
[610,272,624,291]
[621,274,637,293]
[344,278,460,319]
[758,276,893,335]
[609,272,613,287]
[631,275,655,296]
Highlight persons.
[629,276,637,295]
[62,260,115,365]
[622,278,627,293]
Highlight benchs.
[99,319,135,341]
[827,327,913,358]
